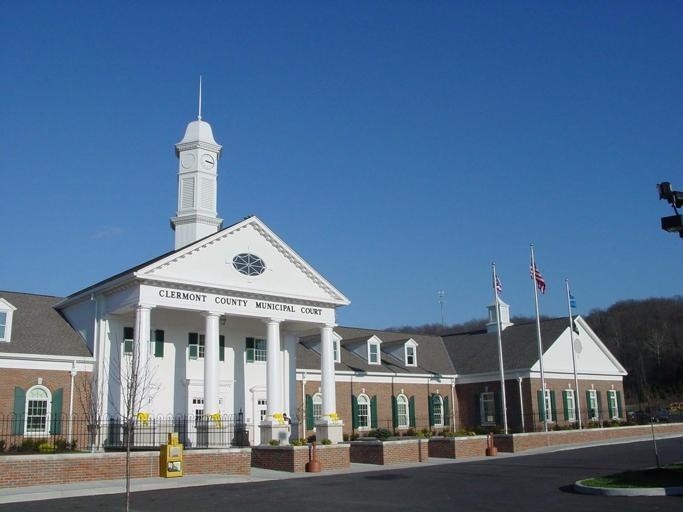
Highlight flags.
[530,255,545,295]
[492,269,502,292]
[569,288,576,308]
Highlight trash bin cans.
[161,431,183,478]
